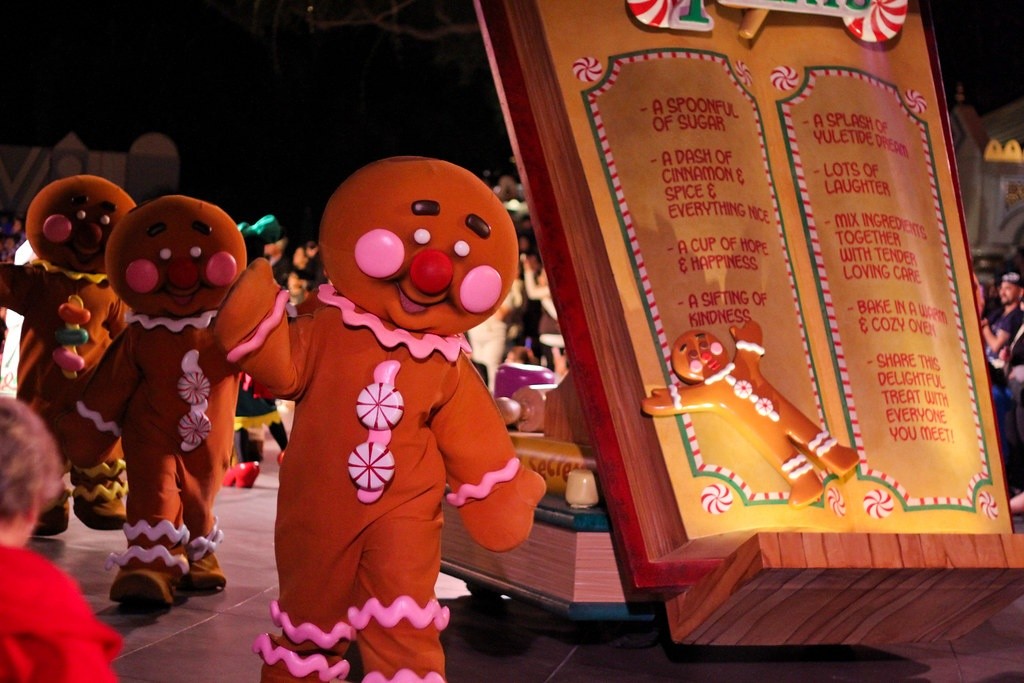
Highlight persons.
[0,395,125,683]
[219,152,547,683]
[636,322,860,508]
[57,196,242,615]
[973,268,1024,513]
[235,227,329,445]
[0,211,31,381]
[463,225,569,396]
[222,387,291,490]
[0,175,139,535]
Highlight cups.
[565,470,599,506]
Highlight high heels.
[223,460,260,487]
[277,450,285,465]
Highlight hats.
[1002,272,1024,288]
